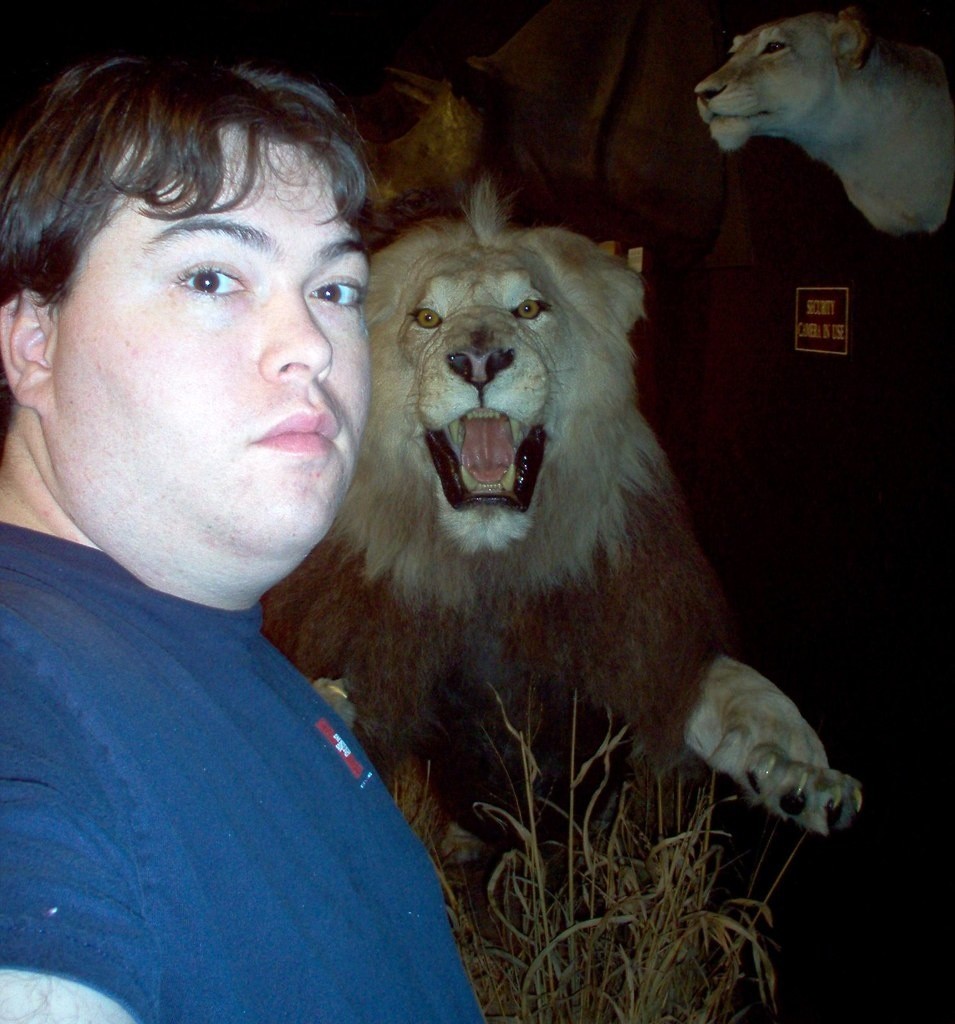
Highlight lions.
[263,175,867,869]
[692,0,955,238]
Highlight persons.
[0,61,488,1023]
